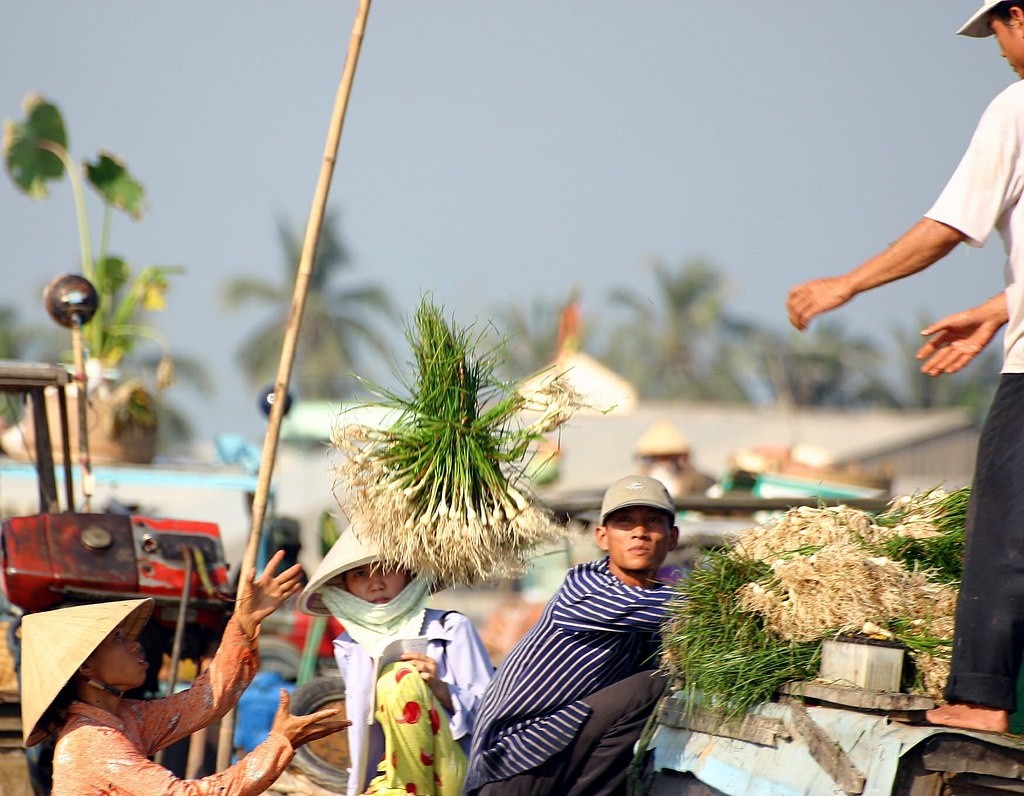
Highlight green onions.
[640,480,970,733]
[324,289,586,582]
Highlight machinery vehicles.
[1,277,1024,796]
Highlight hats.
[955,1,1002,39]
[295,515,459,617]
[599,475,675,527]
[20,596,156,749]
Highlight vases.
[24,364,158,466]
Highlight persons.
[465,475,694,796]
[20,548,352,796]
[786,0,1024,733]
[298,521,494,796]
